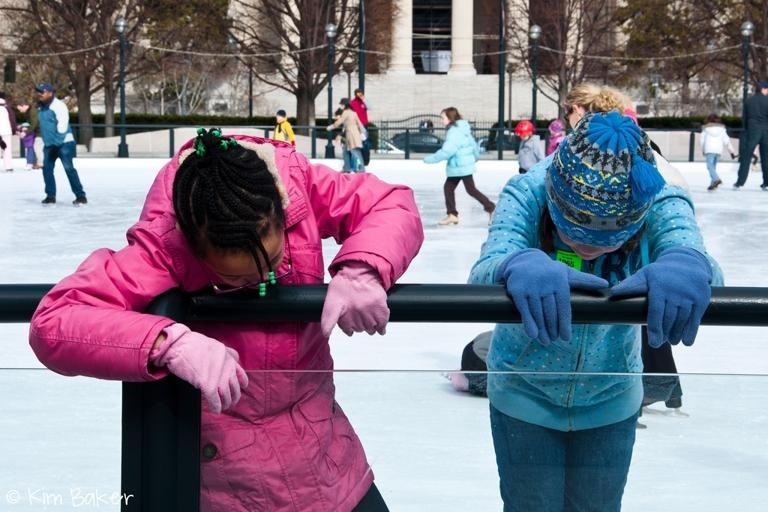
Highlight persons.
[325,90,369,173]
[2,80,87,205]
[700,114,735,190]
[423,107,495,226]
[27,126,426,512]
[468,112,723,512]
[273,110,296,146]
[734,82,768,189]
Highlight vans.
[487,118,553,151]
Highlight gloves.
[149,323,250,416]
[495,247,610,346]
[609,245,713,349]
[321,262,391,340]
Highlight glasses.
[205,219,293,293]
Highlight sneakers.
[41,197,56,204]
[487,206,496,226]
[707,180,723,190]
[25,163,44,171]
[436,214,460,226]
[760,185,768,192]
[730,183,744,190]
[73,197,88,205]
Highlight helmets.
[514,121,539,136]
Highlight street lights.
[739,13,755,161]
[114,14,131,157]
[324,22,337,158]
[599,51,613,87]
[342,53,354,100]
[506,62,516,145]
[527,21,543,129]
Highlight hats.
[754,80,768,89]
[545,111,660,248]
[20,122,30,128]
[339,98,351,105]
[35,82,58,94]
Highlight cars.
[475,137,489,152]
[390,132,444,153]
[368,136,405,155]
